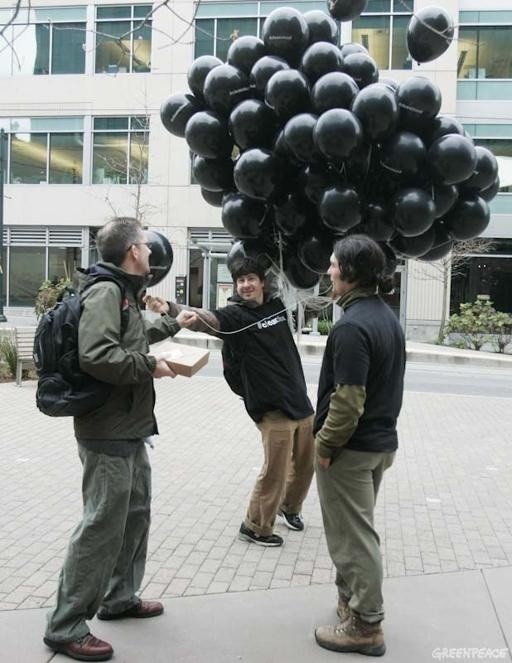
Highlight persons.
[310,234,408,655]
[142,255,315,543]
[47,212,199,660]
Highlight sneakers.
[315,594,386,656]
[238,521,283,546]
[277,505,305,531]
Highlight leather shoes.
[98,600,163,621]
[43,628,113,662]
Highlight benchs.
[14,325,36,388]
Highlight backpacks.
[33,272,130,417]
[222,304,247,399]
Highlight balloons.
[143,229,173,285]
[327,3,366,19]
[159,6,500,290]
